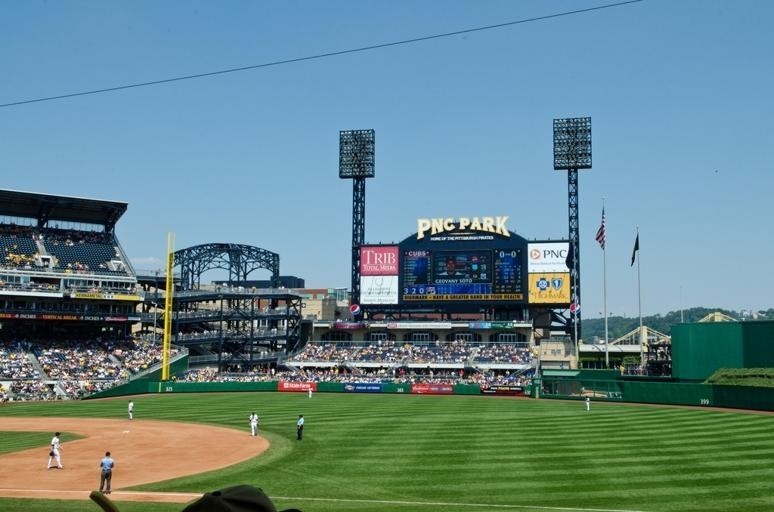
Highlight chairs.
[0,232,134,289]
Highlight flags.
[594,206,606,250]
[629,232,639,267]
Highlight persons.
[0,224,538,402]
[296,414,304,441]
[585,395,590,411]
[126,399,133,421]
[612,336,671,378]
[46,431,63,468]
[248,411,258,437]
[99,451,113,495]
